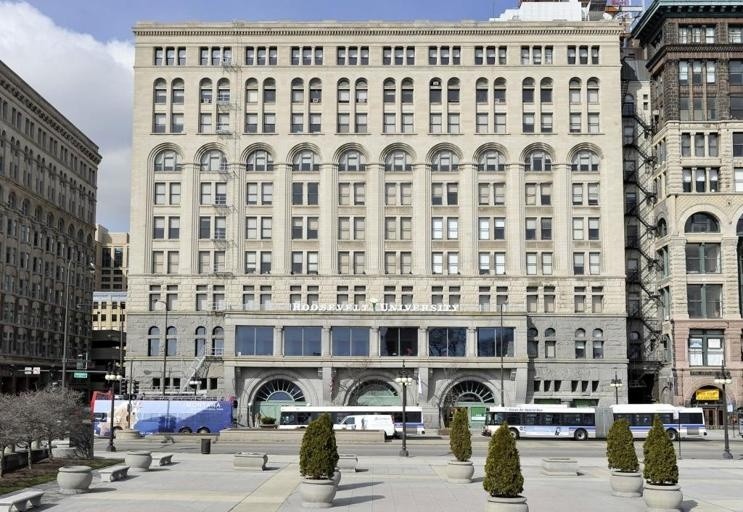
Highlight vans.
[338,415,396,440]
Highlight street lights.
[609,372,623,406]
[714,363,735,464]
[393,358,414,457]
[104,372,124,453]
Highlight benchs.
[0,490,47,512]
[98,465,130,483]
[150,454,174,467]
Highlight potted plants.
[297,410,341,510]
[443,406,529,512]
[604,417,685,512]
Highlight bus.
[91,397,235,439]
[276,406,424,440]
[484,403,708,443]
